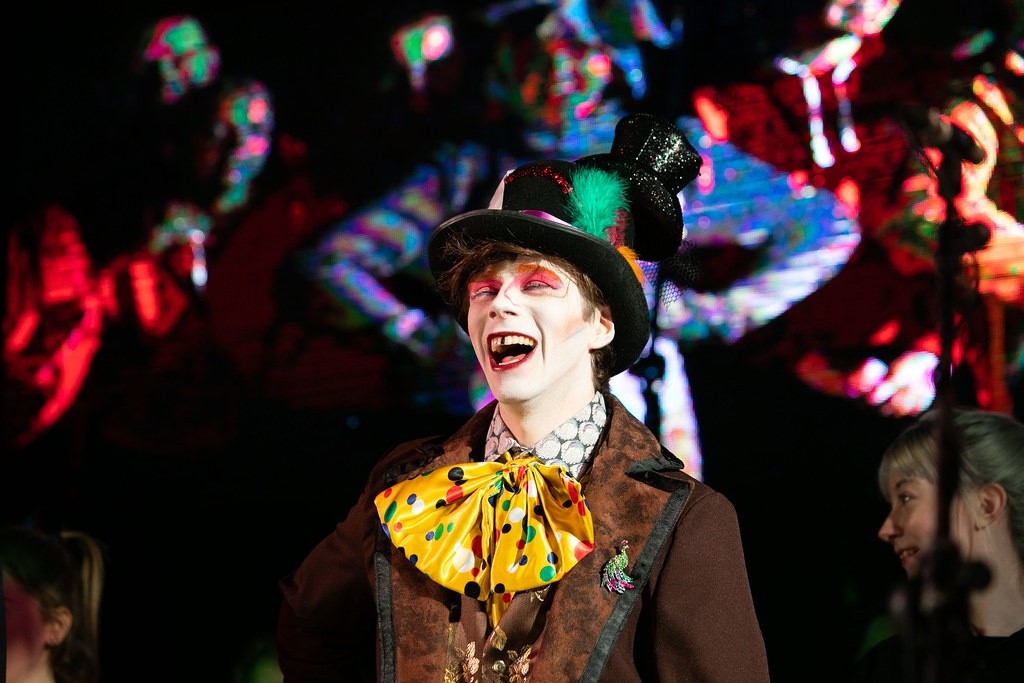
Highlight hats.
[427,113,704,381]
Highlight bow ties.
[373,454,594,629]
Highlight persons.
[847,408,1024,683]
[0,526,105,683]
[276,159,771,683]
[0,0,1024,483]
